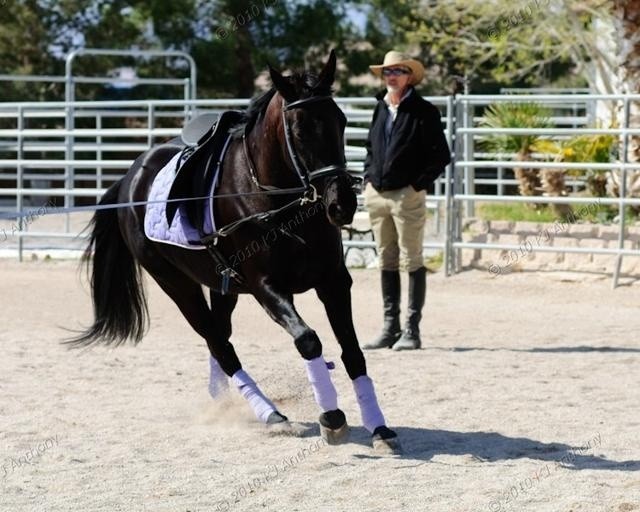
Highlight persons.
[234,43,256,110]
[361,51,451,352]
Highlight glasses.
[382,68,411,75]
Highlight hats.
[369,50,424,86]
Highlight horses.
[54,47,404,456]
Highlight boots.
[362,266,427,351]
